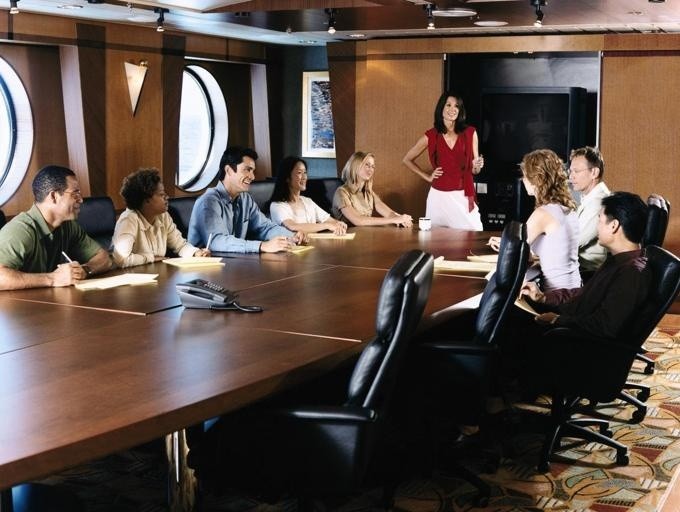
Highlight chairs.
[583,194,671,422]
[414,220,530,506]
[322,179,341,205]
[511,242,680,475]
[166,197,197,240]
[188,249,434,512]
[246,181,279,219]
[77,197,116,250]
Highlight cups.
[418,217,431,230]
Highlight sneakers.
[455,407,512,434]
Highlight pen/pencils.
[206,233,213,250]
[61,251,73,262]
[518,273,528,300]
[394,213,414,221]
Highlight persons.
[522,149,586,290]
[265,157,348,236]
[187,145,309,253]
[460,190,653,438]
[567,147,612,283]
[331,151,413,229]
[112,168,211,269]
[402,90,485,232]
[0,166,112,292]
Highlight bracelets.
[82,266,92,276]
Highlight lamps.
[324,9,340,34]
[422,4,437,30]
[154,7,169,32]
[530,0,547,28]
[7,0,21,14]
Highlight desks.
[0,297,145,355]
[0,256,334,315]
[0,316,363,512]
[208,222,543,279]
[149,265,488,349]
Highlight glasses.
[567,166,593,175]
[362,162,376,170]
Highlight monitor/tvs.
[473,85,588,169]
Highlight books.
[514,296,540,315]
[163,257,226,269]
[467,255,499,263]
[288,246,312,254]
[434,256,497,273]
[309,232,356,240]
[74,272,160,292]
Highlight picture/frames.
[296,70,338,160]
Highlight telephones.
[175,278,239,309]
